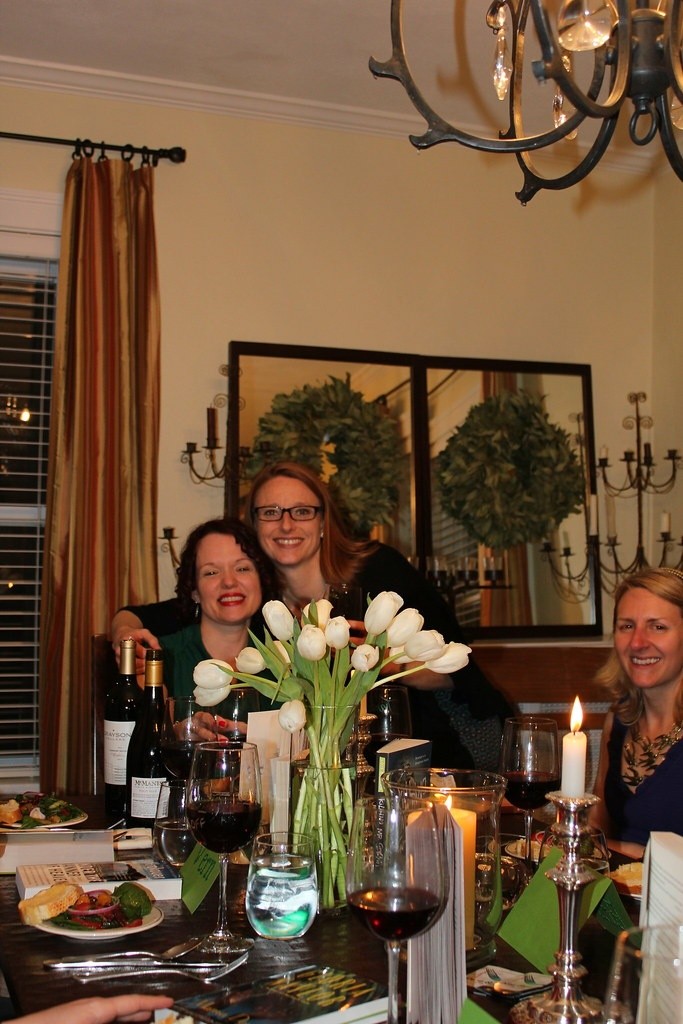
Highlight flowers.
[194,591,472,910]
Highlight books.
[15,859,183,901]
[151,964,402,1024]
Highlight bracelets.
[399,664,406,681]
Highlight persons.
[0,995,174,1024]
[111,459,519,786]
[96,516,284,781]
[581,567,683,868]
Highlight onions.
[67,902,120,915]
[84,890,109,896]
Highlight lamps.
[367,0,683,206]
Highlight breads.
[607,862,643,894]
[18,882,80,925]
[0,799,22,824]
[519,842,552,858]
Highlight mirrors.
[224,339,603,643]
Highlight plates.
[504,840,540,863]
[603,872,641,902]
[2,812,89,828]
[36,904,163,940]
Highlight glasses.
[253,505,322,522]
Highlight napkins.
[113,828,153,850]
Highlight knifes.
[43,960,247,968]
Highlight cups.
[364,684,412,767]
[603,924,683,1024]
[380,769,508,967]
[153,780,197,866]
[474,834,528,911]
[246,833,318,940]
[538,825,609,882]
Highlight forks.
[73,952,248,983]
[53,817,128,832]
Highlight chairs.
[511,702,612,796]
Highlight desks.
[0,795,640,1024]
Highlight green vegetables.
[17,793,84,831]
[50,882,153,930]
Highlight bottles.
[104,640,143,827]
[125,649,179,827]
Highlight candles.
[407,795,476,949]
[644,442,650,457]
[604,494,616,537]
[589,494,598,536]
[543,533,551,543]
[562,532,569,548]
[206,407,216,440]
[600,445,608,458]
[428,570,502,580]
[660,511,670,532]
[561,695,587,797]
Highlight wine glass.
[346,796,450,1023]
[499,715,560,895]
[161,695,218,800]
[216,688,260,800]
[186,744,263,952]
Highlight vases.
[292,759,360,916]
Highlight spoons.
[113,830,127,841]
[62,939,202,961]
